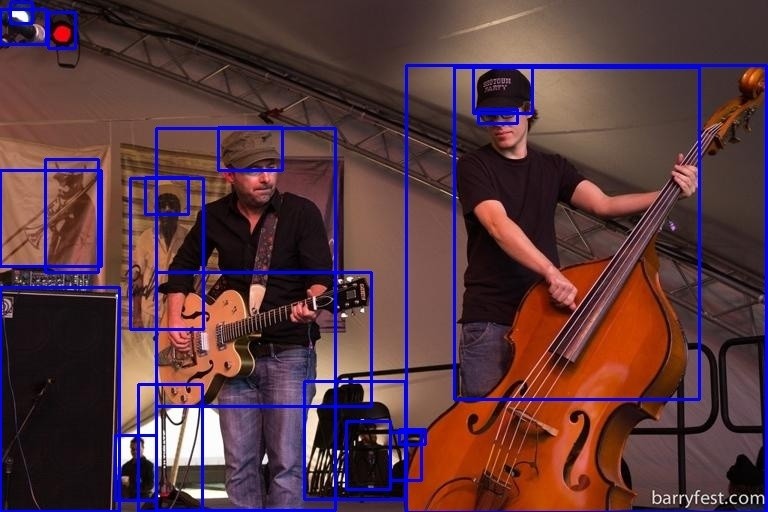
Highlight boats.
[0,288,120,512]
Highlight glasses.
[10,3,32,24]
[48,13,76,48]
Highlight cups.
[479,110,518,123]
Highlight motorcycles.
[138,385,202,512]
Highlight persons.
[454,68,700,400]
[46,160,98,273]
[347,421,391,490]
[130,179,204,330]
[116,436,156,500]
[391,433,422,481]
[156,129,336,510]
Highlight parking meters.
[0,171,101,266]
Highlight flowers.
[0,9,47,45]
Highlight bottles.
[219,128,283,170]
[473,67,533,113]
[146,178,188,214]
[400,430,425,444]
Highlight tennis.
[407,65,766,512]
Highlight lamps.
[305,382,406,500]
